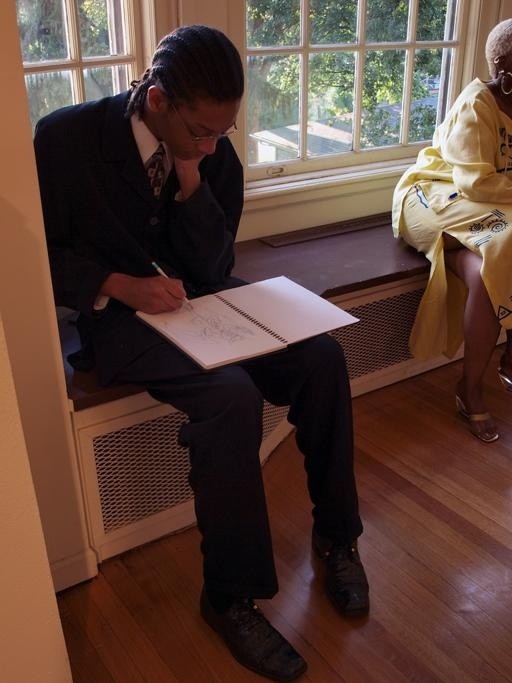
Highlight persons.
[392,19,512,443]
[34,25,369,683]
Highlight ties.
[144,141,167,200]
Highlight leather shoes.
[200,586,308,681]
[311,522,370,618]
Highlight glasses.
[173,105,240,143]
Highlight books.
[136,275,361,370]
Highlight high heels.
[497,368,512,393]
[455,391,499,443]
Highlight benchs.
[62,214,508,563]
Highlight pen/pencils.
[151,261,193,309]
